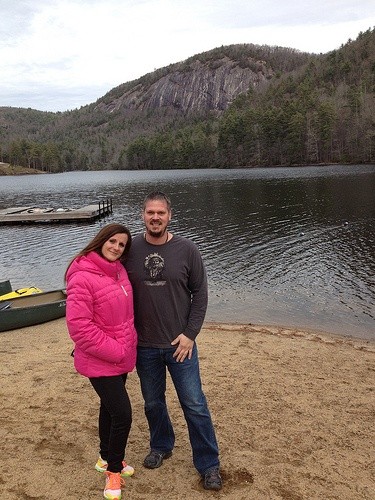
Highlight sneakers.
[142,448,172,469]
[103,472,122,499]
[201,470,222,490]
[95,457,134,478]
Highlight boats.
[0,278,68,332]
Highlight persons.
[64,224,137,500]
[121,191,223,492]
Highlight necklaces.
[145,231,169,243]
